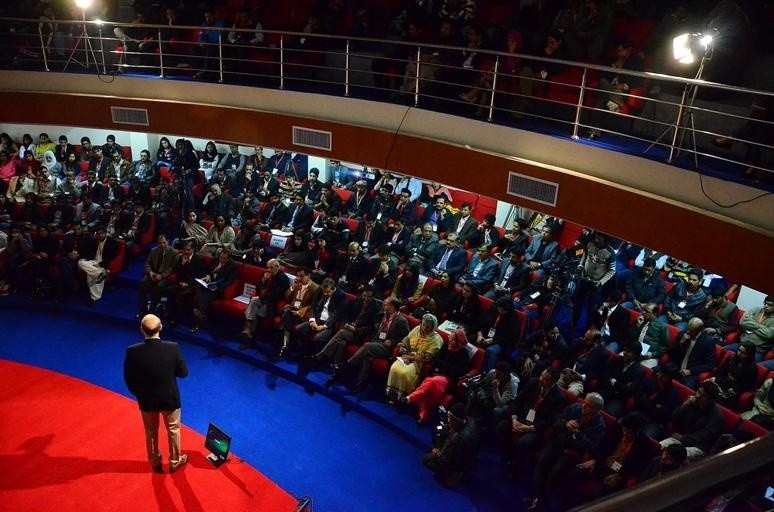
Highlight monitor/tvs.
[204,422,232,467]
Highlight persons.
[124,314,188,472]
[277,3,345,91]
[509,2,747,139]
[352,2,509,115]
[1,2,268,83]
[713,96,772,177]
[242,159,453,405]
[1,134,295,332]
[424,204,773,512]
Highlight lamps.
[643,23,724,170]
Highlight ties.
[639,321,650,343]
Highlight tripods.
[640,52,705,171]
[62,11,99,75]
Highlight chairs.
[0,143,774,512]
[747,97,768,123]
[10,0,656,120]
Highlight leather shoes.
[170,454,187,471]
